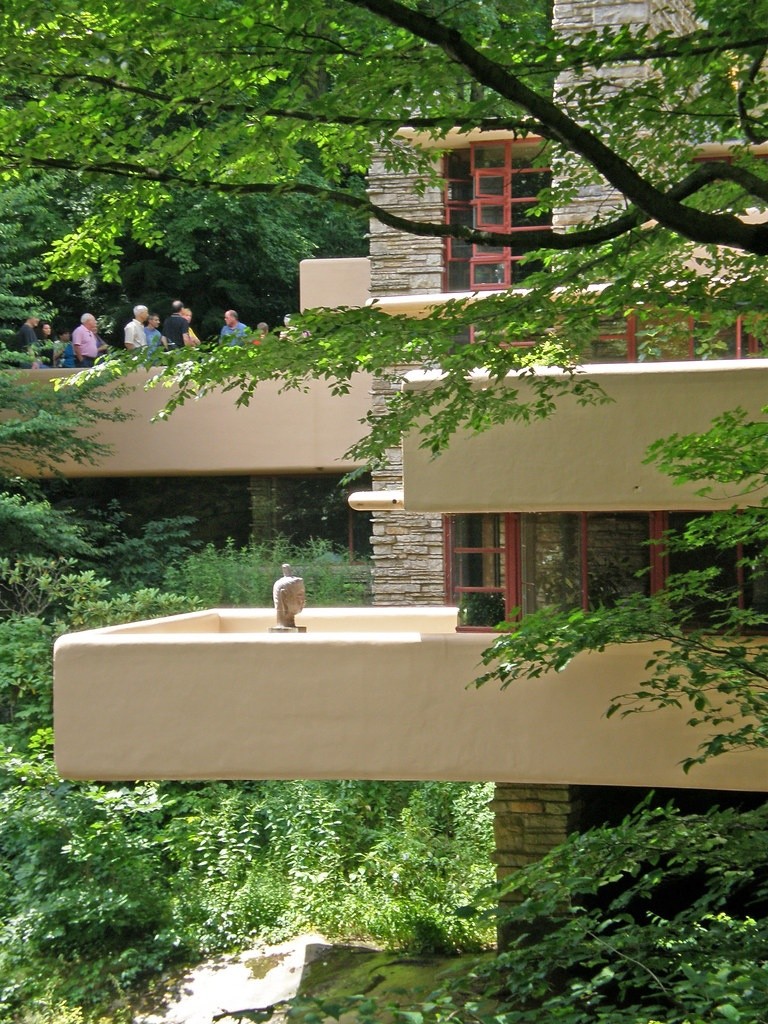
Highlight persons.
[9,310,109,370]
[219,310,247,346]
[253,322,268,345]
[273,563,307,628]
[123,301,201,366]
[279,314,310,341]
